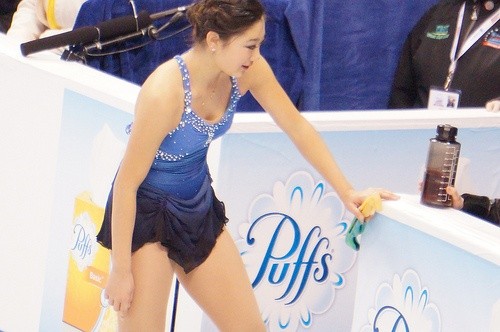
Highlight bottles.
[422,125,461,207]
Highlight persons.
[388,0,500,115]
[96,0,400,332]
[7,0,87,55]
[447,96,455,108]
[418,181,500,227]
